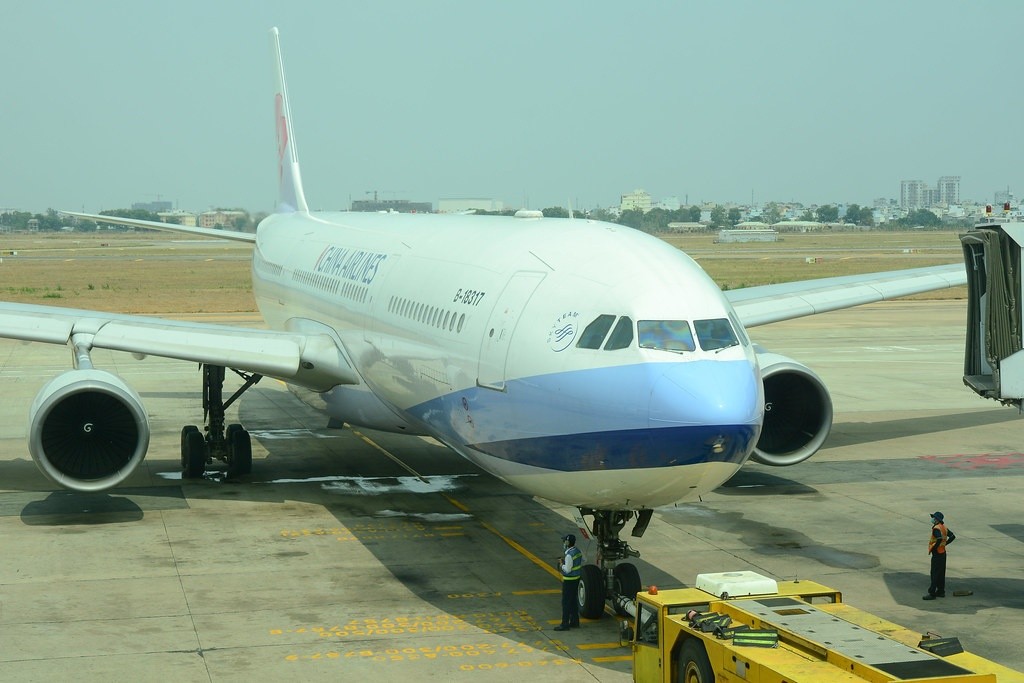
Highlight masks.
[930,518,936,524]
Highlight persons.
[922,511,956,600]
[553,534,582,631]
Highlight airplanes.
[0,22,970,618]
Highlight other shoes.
[569,623,579,627]
[923,595,935,600]
[554,627,569,631]
[934,593,945,597]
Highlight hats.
[930,512,944,522]
[561,534,576,548]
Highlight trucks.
[621,570,1024,683]
